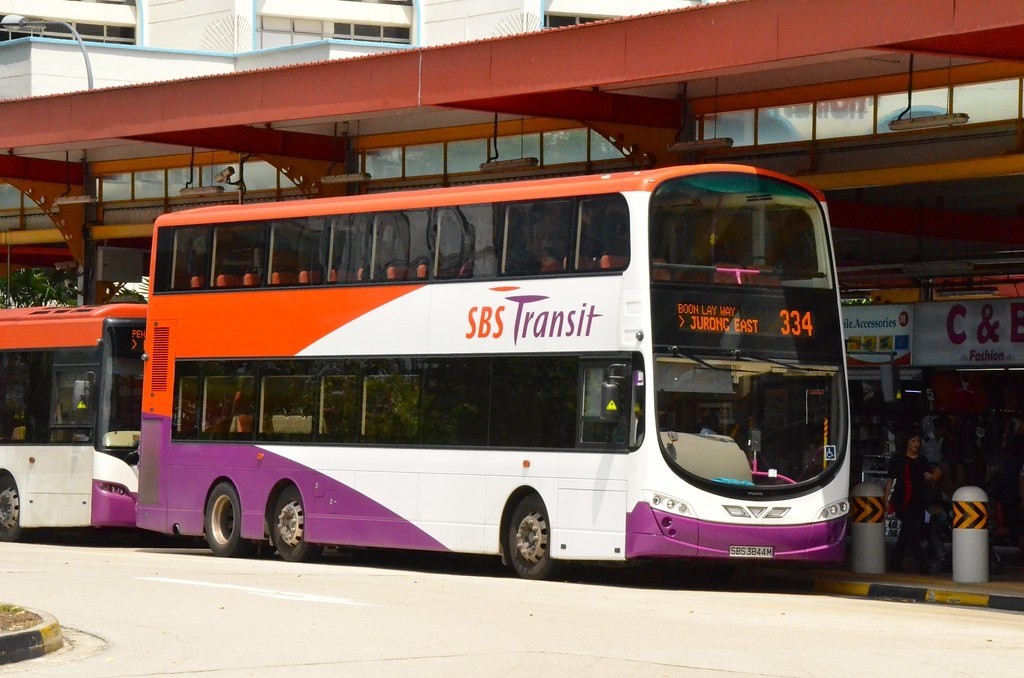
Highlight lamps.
[888,54,970,132]
[54,151,98,204]
[179,148,226,196]
[478,112,538,172]
[666,83,734,152]
[321,123,372,184]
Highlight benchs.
[387,267,416,279]
[271,270,298,283]
[600,255,671,282]
[299,270,321,282]
[190,276,210,288]
[244,273,262,285]
[237,413,268,432]
[216,274,244,287]
[331,269,357,281]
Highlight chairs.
[306,406,328,434]
[287,407,308,433]
[271,408,293,433]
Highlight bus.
[135,145,904,582]
[0,286,172,542]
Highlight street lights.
[0,13,94,91]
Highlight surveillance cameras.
[213,166,234,183]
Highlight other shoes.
[920,565,933,575]
[886,564,910,573]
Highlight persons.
[919,416,952,463]
[885,431,933,576]
